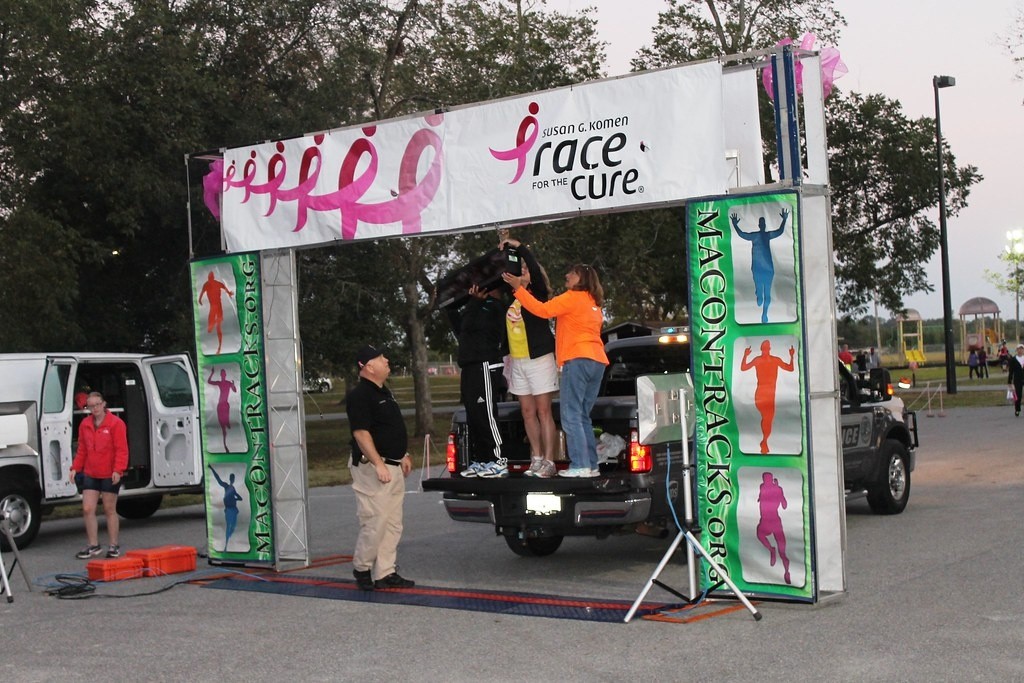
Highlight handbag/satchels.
[1006,384,1018,404]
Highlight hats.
[1017,344,1024,348]
[354,344,389,371]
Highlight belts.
[381,457,400,466]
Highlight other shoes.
[1015,409,1019,417]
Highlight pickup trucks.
[421,326,920,557]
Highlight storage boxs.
[127,544,197,577]
[86,557,143,581]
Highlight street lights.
[1004,229,1024,343]
[933,74,957,394]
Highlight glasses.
[86,401,103,410]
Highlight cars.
[303,378,333,394]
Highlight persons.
[968,346,988,379]
[346,345,416,589]
[445,238,609,478]
[998,339,1012,369]
[1009,344,1024,416]
[838,344,880,374]
[69,392,128,559]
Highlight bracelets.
[405,452,411,456]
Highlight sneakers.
[107,545,120,558]
[353,564,375,590]
[478,461,508,478]
[373,571,416,589]
[558,464,602,478]
[533,461,558,479]
[524,457,544,478]
[76,545,102,558]
[460,459,489,476]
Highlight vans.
[0,353,204,553]
